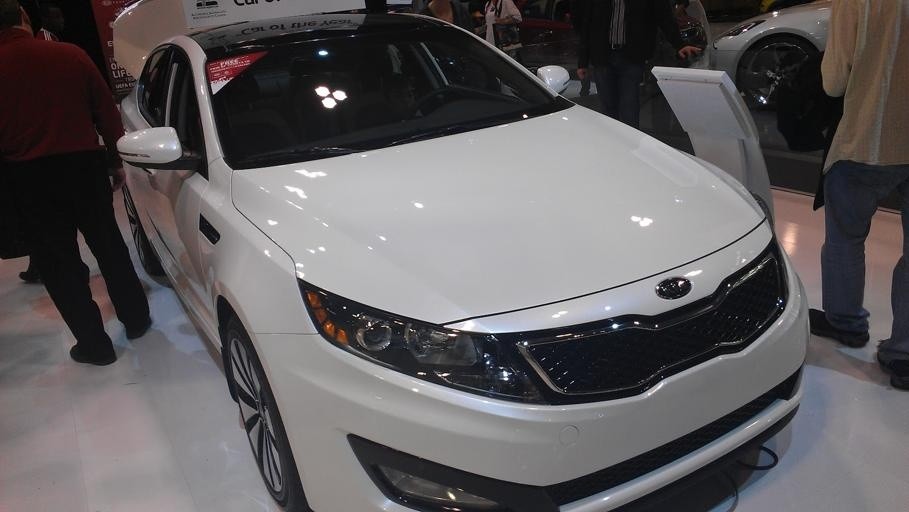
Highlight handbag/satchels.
[492,22,521,47]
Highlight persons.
[416,0,523,96]
[565,0,708,150]
[807,0,909,394]
[1,0,152,365]
[36,6,65,42]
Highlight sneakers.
[70,316,151,365]
[876,344,909,391]
[808,309,870,349]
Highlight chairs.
[220,56,420,158]
